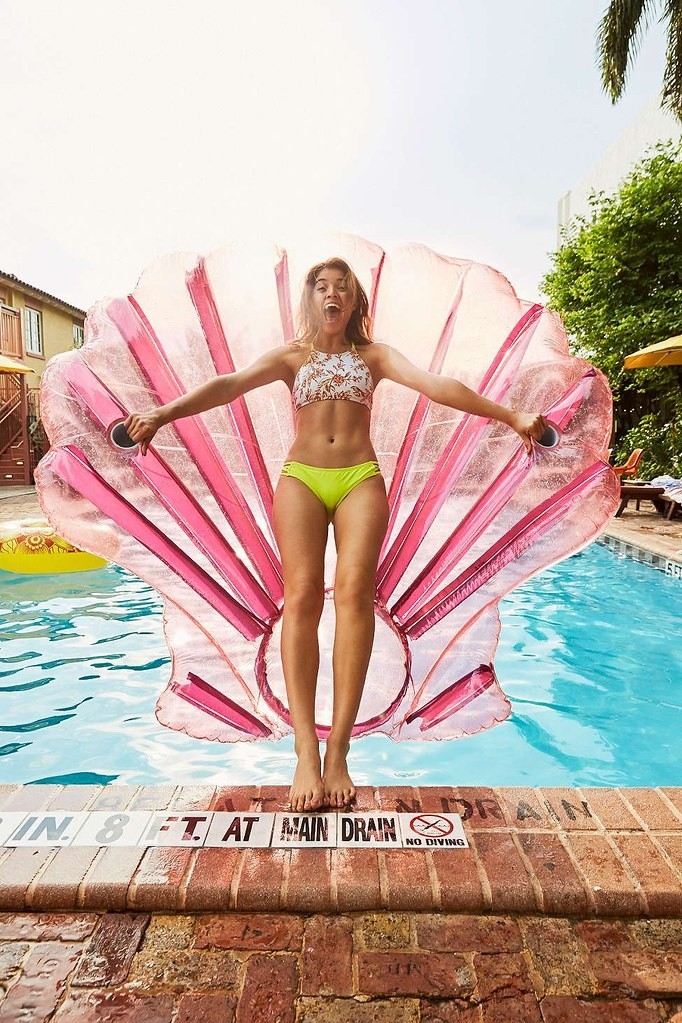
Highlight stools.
[659,495,682,520]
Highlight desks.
[616,486,665,517]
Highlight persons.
[124,257,547,813]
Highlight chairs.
[613,448,645,486]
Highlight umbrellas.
[624,335,682,370]
[0,355,37,375]
